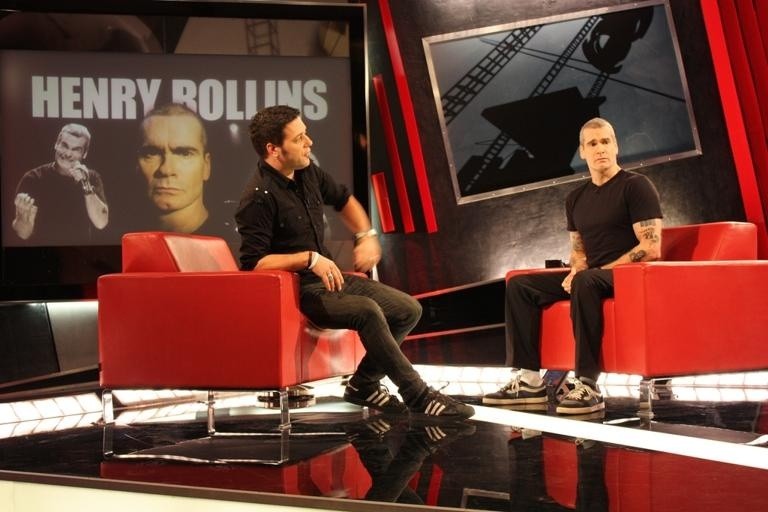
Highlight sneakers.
[556,386,605,414]
[344,414,397,442]
[482,378,549,405]
[344,384,406,414]
[410,387,474,420]
[409,422,476,454]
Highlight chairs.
[100,231,365,466]
[100,437,374,500]
[510,428,768,512]
[508,222,768,419]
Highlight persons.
[483,112,664,415]
[234,107,473,419]
[507,424,610,512]
[11,121,108,243]
[349,420,476,506]
[134,105,220,234]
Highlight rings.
[326,273,333,280]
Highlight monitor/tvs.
[421,0,703,207]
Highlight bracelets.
[303,249,320,271]
[350,228,379,242]
